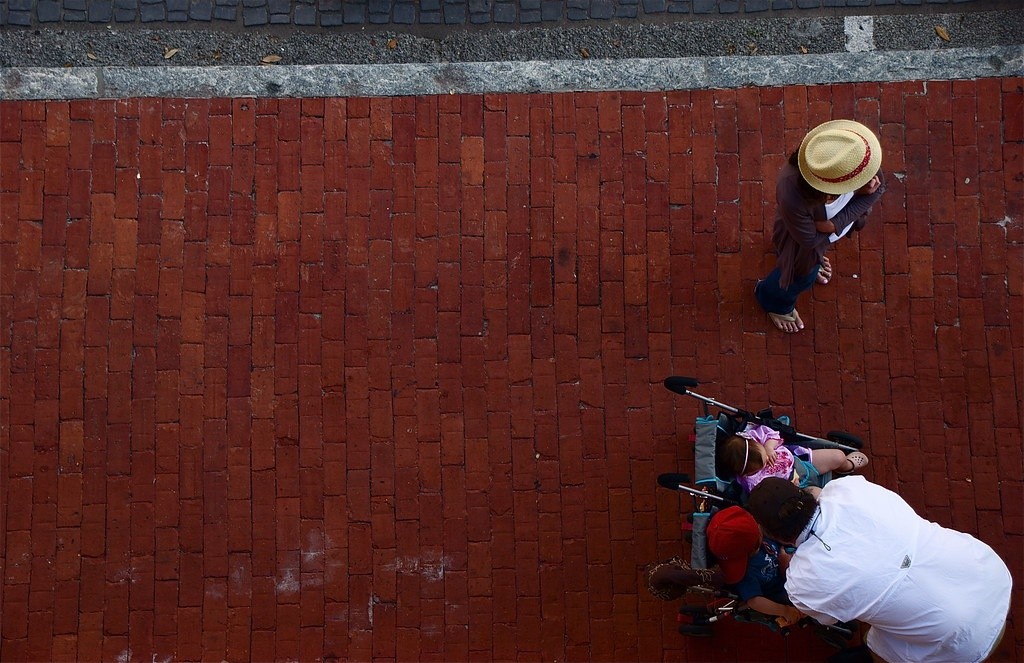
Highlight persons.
[707,505,808,624]
[754,120,886,333]
[724,425,868,491]
[748,475,1013,663]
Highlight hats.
[706,505,761,585]
[747,476,801,532]
[798,118,882,194]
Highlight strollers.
[652,375,869,645]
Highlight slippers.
[754,279,804,333]
[836,451,868,474]
[815,254,832,284]
